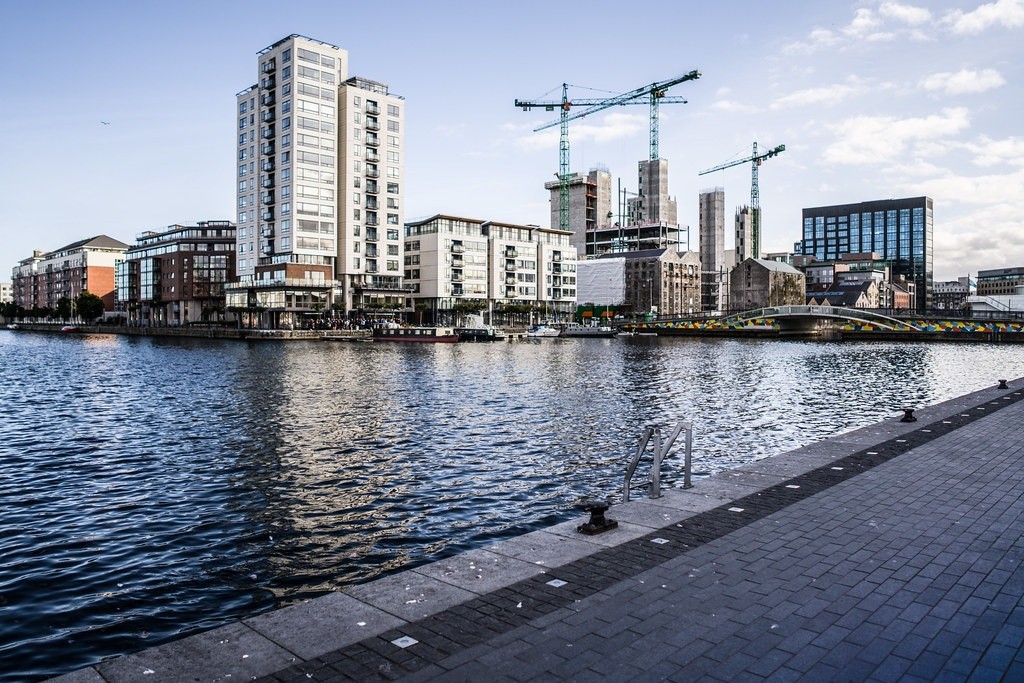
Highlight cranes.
[694,141,787,260]
[530,69,702,223]
[515,84,688,230]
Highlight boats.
[454,328,506,341]
[528,327,560,338]
[7,324,18,330]
[369,327,459,343]
[62,325,77,332]
[562,325,618,339]
